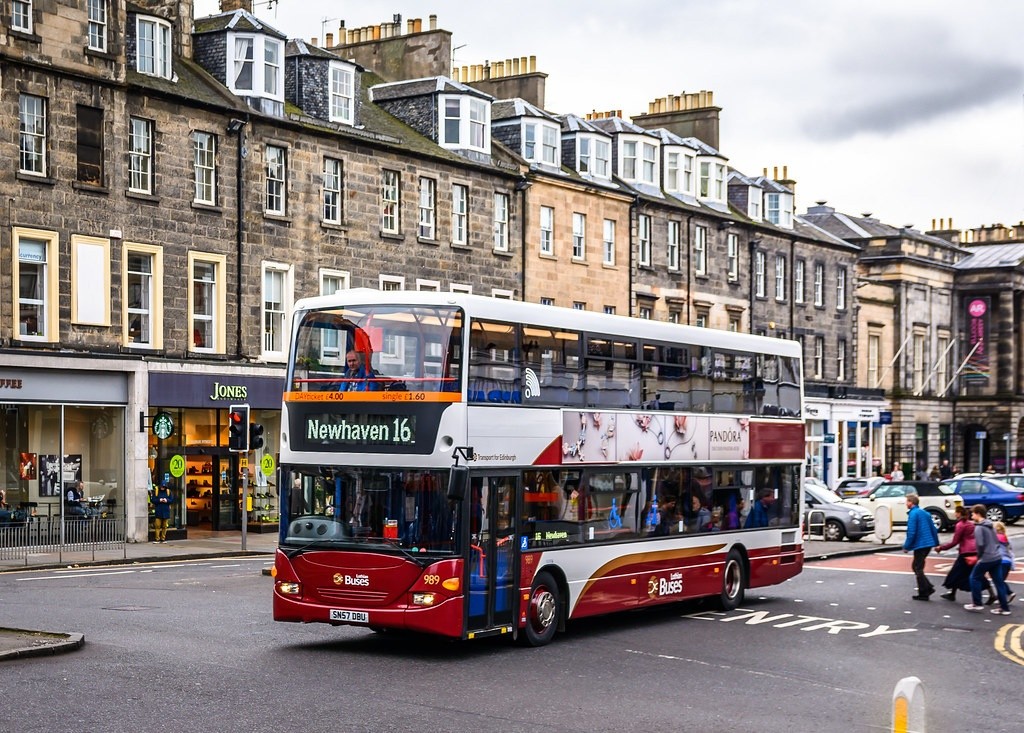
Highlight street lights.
[1003,433,1017,482]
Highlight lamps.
[717,222,735,232]
[226,118,247,136]
[514,181,533,194]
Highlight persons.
[650,486,778,538]
[930,466,940,481]
[339,351,380,392]
[67,481,89,534]
[940,459,953,481]
[963,504,1011,615]
[989,522,1015,604]
[985,463,995,474]
[950,462,962,478]
[934,506,997,605]
[152,478,173,544]
[890,464,904,482]
[903,493,939,601]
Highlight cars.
[950,473,1024,488]
[805,477,829,490]
[844,481,964,532]
[943,479,1024,522]
[805,483,876,542]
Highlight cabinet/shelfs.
[162,446,278,534]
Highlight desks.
[79,500,103,542]
[39,501,60,544]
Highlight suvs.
[834,477,888,498]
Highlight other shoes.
[153,539,160,544]
[187,463,280,523]
[913,595,928,600]
[1007,593,1015,603]
[161,540,167,544]
[942,594,956,601]
[990,608,1011,615]
[986,596,998,605]
[964,604,985,610]
[928,588,935,596]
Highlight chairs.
[19,498,118,545]
[405,374,740,413]
[890,489,901,498]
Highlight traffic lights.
[228,405,249,453]
[250,423,264,449]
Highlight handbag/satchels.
[965,555,978,565]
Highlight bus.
[273,287,806,644]
